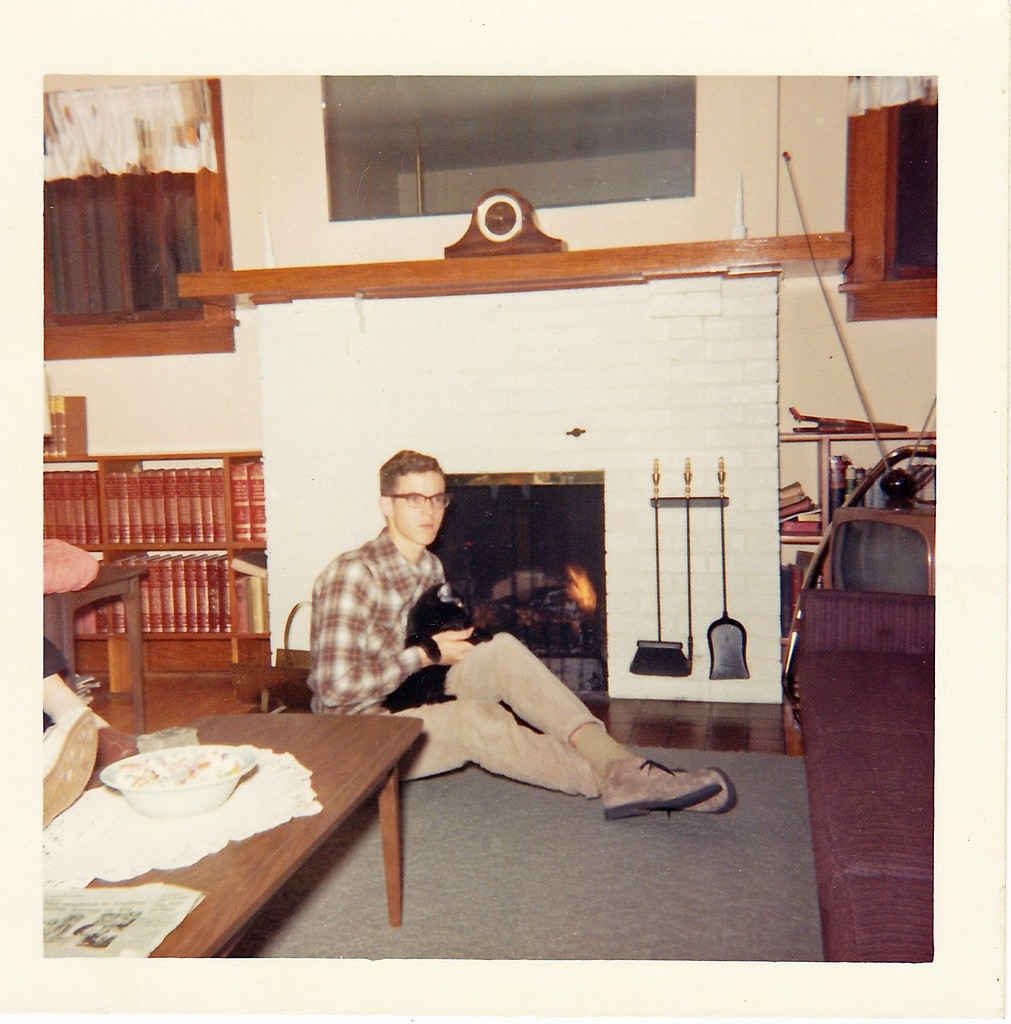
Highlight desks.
[43,713,425,958]
[44,564,147,739]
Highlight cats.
[381,581,475,714]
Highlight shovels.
[706,456,751,681]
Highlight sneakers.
[602,759,722,819]
[637,761,736,820]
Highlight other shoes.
[95,727,140,765]
[43,706,98,827]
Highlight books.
[829,454,936,522]
[43,456,266,545]
[780,551,822,727]
[43,396,89,458]
[73,549,270,635]
[779,482,822,536]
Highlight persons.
[43,636,138,828]
[309,449,738,822]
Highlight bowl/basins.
[98,743,260,817]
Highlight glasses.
[385,492,453,509]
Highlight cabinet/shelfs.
[44,450,269,692]
[775,432,936,595]
